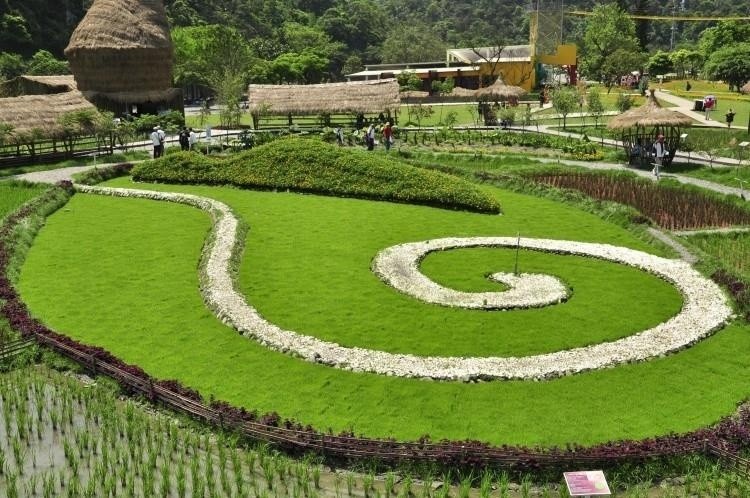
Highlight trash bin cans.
[693,99,704,111]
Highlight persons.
[188,127,196,148]
[158,124,166,156]
[150,126,162,159]
[703,97,715,121]
[383,122,392,151]
[178,126,190,152]
[653,134,670,173]
[725,108,737,129]
[337,127,345,145]
[367,123,375,152]
